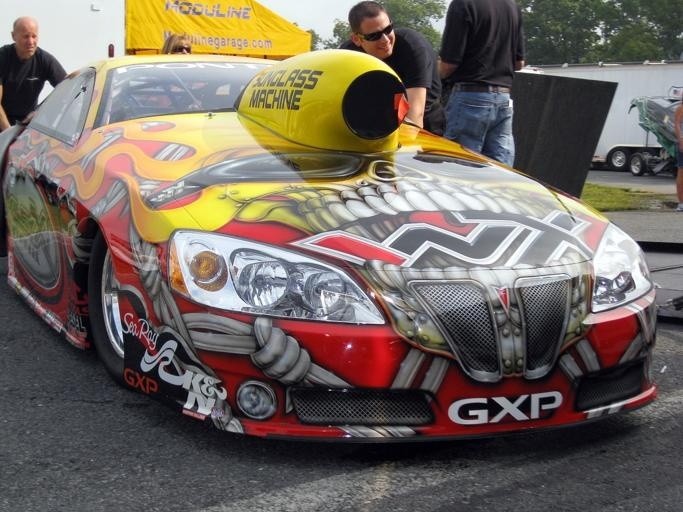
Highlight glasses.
[359,19,394,42]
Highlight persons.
[162,33,192,55]
[339,1,447,136]
[437,0,525,169]
[1,16,68,258]
[674,104,683,212]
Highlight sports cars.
[0,49,662,445]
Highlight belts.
[452,85,511,94]
